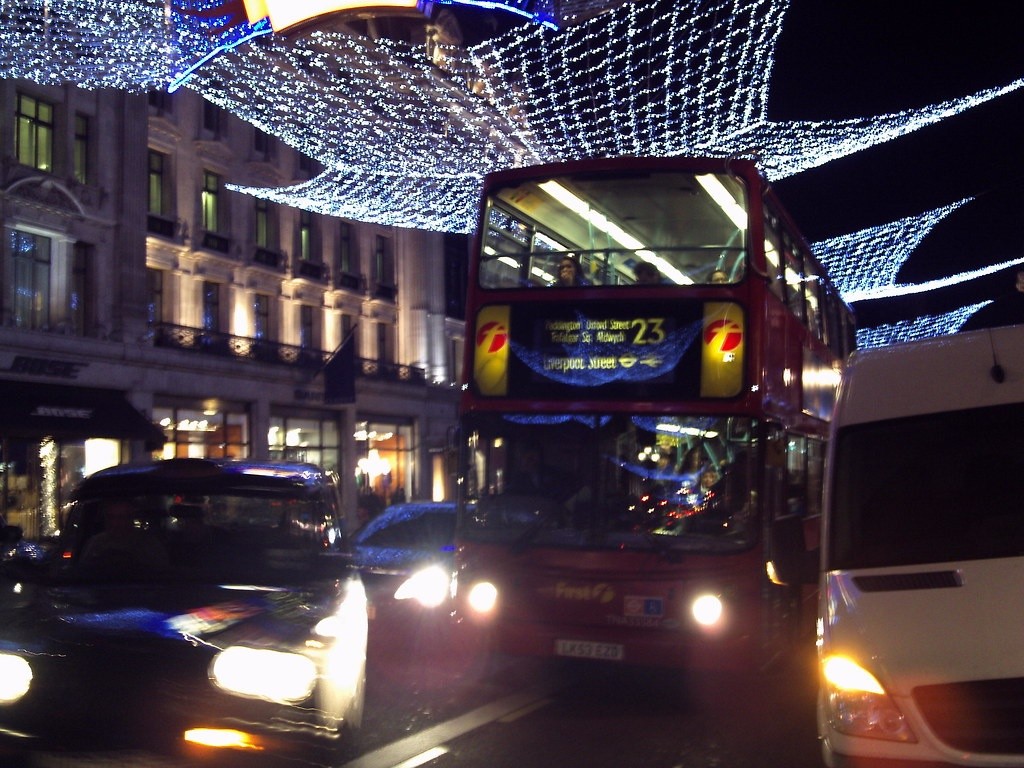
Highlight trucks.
[823,322,1024,767]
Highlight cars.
[0,456,481,750]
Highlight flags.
[324,335,356,406]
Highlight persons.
[697,459,806,517]
[547,257,740,287]
[85,499,147,564]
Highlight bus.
[449,157,861,667]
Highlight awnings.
[0,378,169,443]
